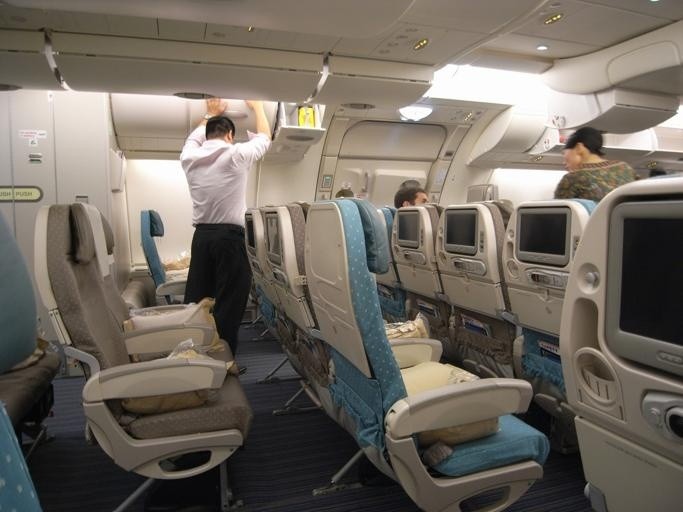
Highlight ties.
[397,211,420,247]
[604,201,683,379]
[245,214,257,256]
[515,206,570,267]
[444,208,478,256]
[266,213,282,264]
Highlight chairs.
[0,174,683,512]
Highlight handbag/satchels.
[203,114,213,120]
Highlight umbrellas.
[240,367,247,373]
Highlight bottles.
[563,126,606,157]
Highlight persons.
[553,126,637,205]
[335,189,354,198]
[400,179,421,190]
[177,96,272,378]
[394,187,430,210]
